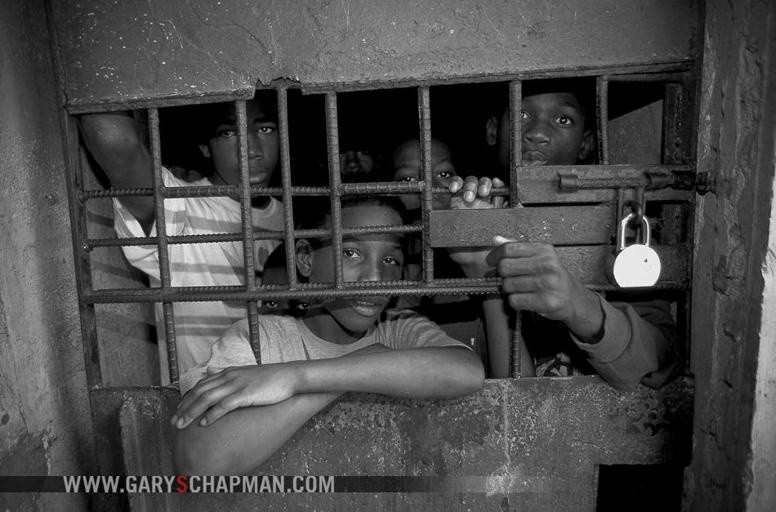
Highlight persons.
[259,241,314,318]
[391,135,537,378]
[80,91,284,386]
[316,125,374,181]
[171,195,484,478]
[486,79,675,391]
